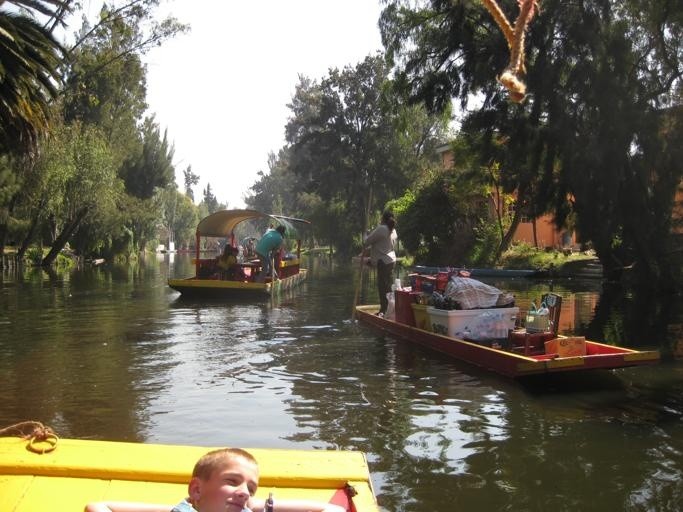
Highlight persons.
[213,243,235,274]
[230,247,238,263]
[253,225,286,279]
[169,445,260,511]
[361,209,398,320]
[242,239,257,263]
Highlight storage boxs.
[544,335,586,358]
[424,305,519,340]
[410,303,436,332]
[394,289,422,328]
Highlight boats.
[0,421,380,512]
[166,209,311,294]
[353,303,661,380]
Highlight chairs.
[510,292,562,356]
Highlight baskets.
[525,302,550,333]
